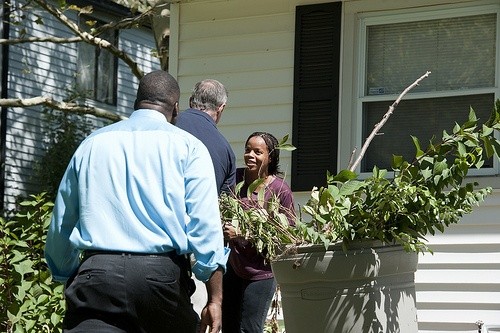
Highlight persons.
[174,79,237,333]
[45,69,231,333]
[221,131,296,333]
